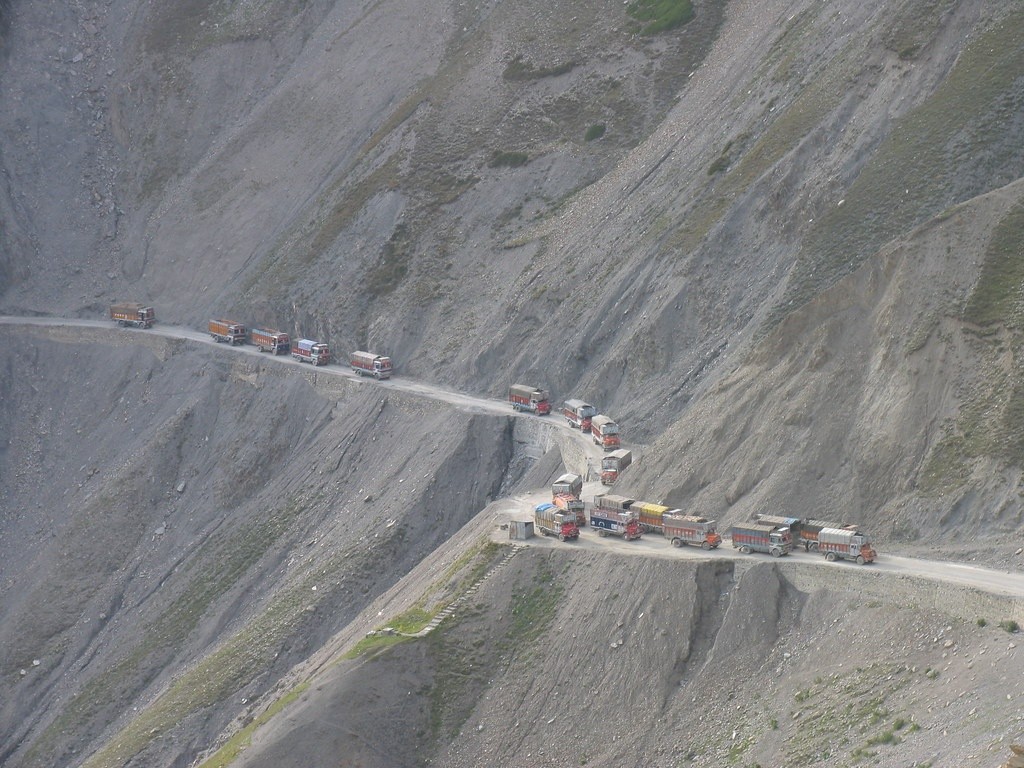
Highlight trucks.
[208,318,248,346]
[109,302,155,329]
[600,448,632,486]
[591,414,621,452]
[508,383,553,416]
[563,399,597,433]
[534,472,878,565]
[290,336,331,366]
[350,351,392,380]
[251,328,290,356]
